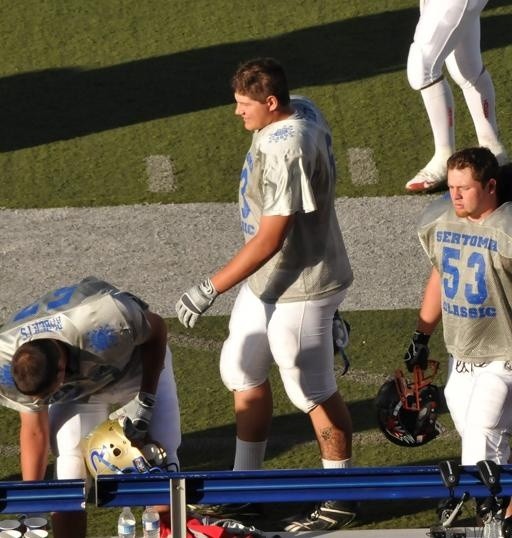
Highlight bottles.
[117,506,136,537]
[140,505,160,537]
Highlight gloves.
[404,331,430,373]
[107,391,157,439]
[174,277,218,329]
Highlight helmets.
[80,418,168,482]
[375,359,442,448]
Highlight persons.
[175,57,356,533]
[407,0,508,192]
[406,146,511,526]
[0,277,182,538]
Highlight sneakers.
[282,498,368,533]
[201,504,265,520]
[490,144,511,168]
[404,162,448,192]
